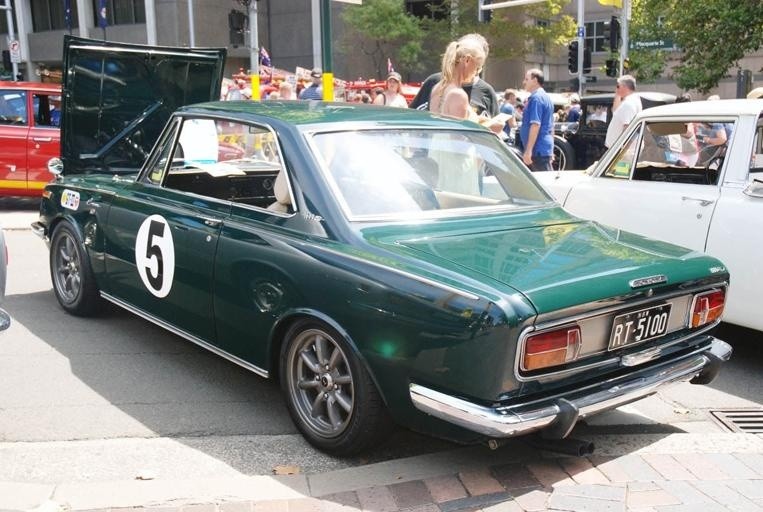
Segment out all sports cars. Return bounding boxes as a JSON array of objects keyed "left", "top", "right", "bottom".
[{"left": 34, "top": 35, "right": 733, "bottom": 456}]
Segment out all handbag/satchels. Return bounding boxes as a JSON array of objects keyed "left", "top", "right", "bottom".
[{"left": 655, "top": 130, "right": 696, "bottom": 152}]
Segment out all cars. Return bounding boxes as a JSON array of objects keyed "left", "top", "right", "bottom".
[
  {"left": 0, "top": 79, "right": 243, "bottom": 199},
  {"left": 510, "top": 92, "right": 677, "bottom": 173}
]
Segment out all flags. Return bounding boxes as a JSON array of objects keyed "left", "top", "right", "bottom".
[
  {"left": 64, "top": 0, "right": 72, "bottom": 30},
  {"left": 259, "top": 46, "right": 271, "bottom": 67},
  {"left": 96, "top": 0, "right": 107, "bottom": 31}
]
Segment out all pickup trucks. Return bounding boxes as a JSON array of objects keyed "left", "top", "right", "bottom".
[{"left": 480, "top": 100, "right": 763, "bottom": 333}]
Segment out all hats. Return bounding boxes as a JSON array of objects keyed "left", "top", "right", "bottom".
[
  {"left": 311, "top": 68, "right": 321, "bottom": 78},
  {"left": 386, "top": 72, "right": 401, "bottom": 81}
]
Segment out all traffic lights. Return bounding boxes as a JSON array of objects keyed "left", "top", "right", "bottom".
[
  {"left": 567, "top": 40, "right": 579, "bottom": 73},
  {"left": 605, "top": 58, "right": 617, "bottom": 78}
]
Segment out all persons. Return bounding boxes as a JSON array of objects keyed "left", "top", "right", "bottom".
[
  {"left": 497, "top": 89, "right": 607, "bottom": 143},
  {"left": 425, "top": 38, "right": 507, "bottom": 197},
  {"left": 604, "top": 74, "right": 642, "bottom": 150},
  {"left": 658, "top": 93, "right": 733, "bottom": 169},
  {"left": 407, "top": 32, "right": 498, "bottom": 197},
  {"left": 520, "top": 68, "right": 555, "bottom": 172},
  {"left": 223, "top": 67, "right": 408, "bottom": 133}
]
